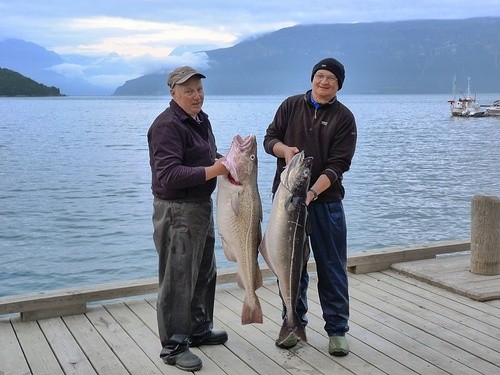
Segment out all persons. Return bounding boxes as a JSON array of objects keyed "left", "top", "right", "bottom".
[
  {"left": 263, "top": 57, "right": 357, "bottom": 355},
  {"left": 147, "top": 66, "right": 229, "bottom": 372}
]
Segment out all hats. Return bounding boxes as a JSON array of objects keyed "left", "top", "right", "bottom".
[
  {"left": 167, "top": 67, "right": 207, "bottom": 88},
  {"left": 311, "top": 58, "right": 345, "bottom": 91}
]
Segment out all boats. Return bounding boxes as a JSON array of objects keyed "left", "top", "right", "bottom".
[{"left": 446, "top": 72, "right": 500, "bottom": 118}]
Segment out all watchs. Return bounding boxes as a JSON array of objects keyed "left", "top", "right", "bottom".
[{"left": 310, "top": 189, "right": 318, "bottom": 200}]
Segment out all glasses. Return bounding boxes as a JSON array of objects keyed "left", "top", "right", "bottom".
[{"left": 314, "top": 73, "right": 338, "bottom": 83}]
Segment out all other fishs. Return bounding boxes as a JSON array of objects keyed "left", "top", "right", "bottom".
[
  {"left": 213, "top": 134, "right": 265, "bottom": 325},
  {"left": 257, "top": 150, "right": 315, "bottom": 345}
]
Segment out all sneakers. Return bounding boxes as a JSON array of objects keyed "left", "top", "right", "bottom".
[
  {"left": 328, "top": 334, "right": 349, "bottom": 355},
  {"left": 175, "top": 354, "right": 202, "bottom": 370},
  {"left": 281, "top": 332, "right": 297, "bottom": 349},
  {"left": 193, "top": 330, "right": 228, "bottom": 347}
]
